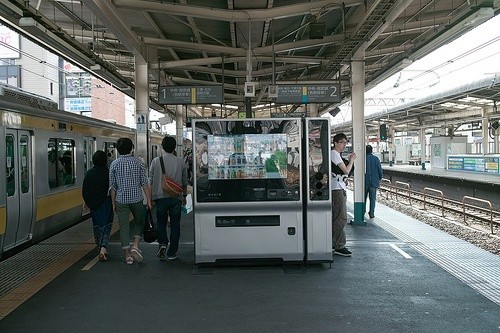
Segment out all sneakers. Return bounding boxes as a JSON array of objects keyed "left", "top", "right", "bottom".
[
  {"left": 332, "top": 246, "right": 335, "bottom": 251},
  {"left": 334, "top": 247, "right": 352, "bottom": 256}
]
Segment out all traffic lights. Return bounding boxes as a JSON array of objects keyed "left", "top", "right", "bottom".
[{"left": 379, "top": 123, "right": 388, "bottom": 141}]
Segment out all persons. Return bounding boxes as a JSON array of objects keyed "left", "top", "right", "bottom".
[
  {"left": 330, "top": 133, "right": 356, "bottom": 256},
  {"left": 365, "top": 145, "right": 382, "bottom": 218},
  {"left": 81, "top": 150, "right": 115, "bottom": 261},
  {"left": 48, "top": 150, "right": 72, "bottom": 187},
  {"left": 149, "top": 136, "right": 187, "bottom": 260},
  {"left": 108, "top": 138, "right": 151, "bottom": 264},
  {"left": 183, "top": 149, "right": 193, "bottom": 186},
  {"left": 255, "top": 152, "right": 262, "bottom": 163}
]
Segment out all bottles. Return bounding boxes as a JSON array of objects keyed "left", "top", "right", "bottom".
[{"left": 211, "top": 140, "right": 266, "bottom": 178}]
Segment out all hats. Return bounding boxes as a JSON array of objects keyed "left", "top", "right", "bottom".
[{"left": 334, "top": 133, "right": 349, "bottom": 142}]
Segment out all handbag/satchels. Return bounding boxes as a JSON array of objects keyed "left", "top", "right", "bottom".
[
  {"left": 144, "top": 207, "right": 157, "bottom": 243},
  {"left": 331, "top": 156, "right": 354, "bottom": 176},
  {"left": 159, "top": 156, "right": 184, "bottom": 196}
]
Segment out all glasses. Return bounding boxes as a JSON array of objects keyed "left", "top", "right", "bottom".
[{"left": 338, "top": 141, "right": 348, "bottom": 144}]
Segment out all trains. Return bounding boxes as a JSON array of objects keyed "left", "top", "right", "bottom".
[{"left": 0, "top": 81, "right": 193, "bottom": 265}]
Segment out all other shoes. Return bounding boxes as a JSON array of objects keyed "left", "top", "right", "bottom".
[
  {"left": 157, "top": 245, "right": 167, "bottom": 258},
  {"left": 370, "top": 215, "right": 375, "bottom": 218},
  {"left": 99, "top": 251, "right": 108, "bottom": 260},
  {"left": 125, "top": 251, "right": 134, "bottom": 264},
  {"left": 131, "top": 245, "right": 144, "bottom": 261},
  {"left": 168, "top": 254, "right": 177, "bottom": 259},
  {"left": 99, "top": 248, "right": 108, "bottom": 253}
]
